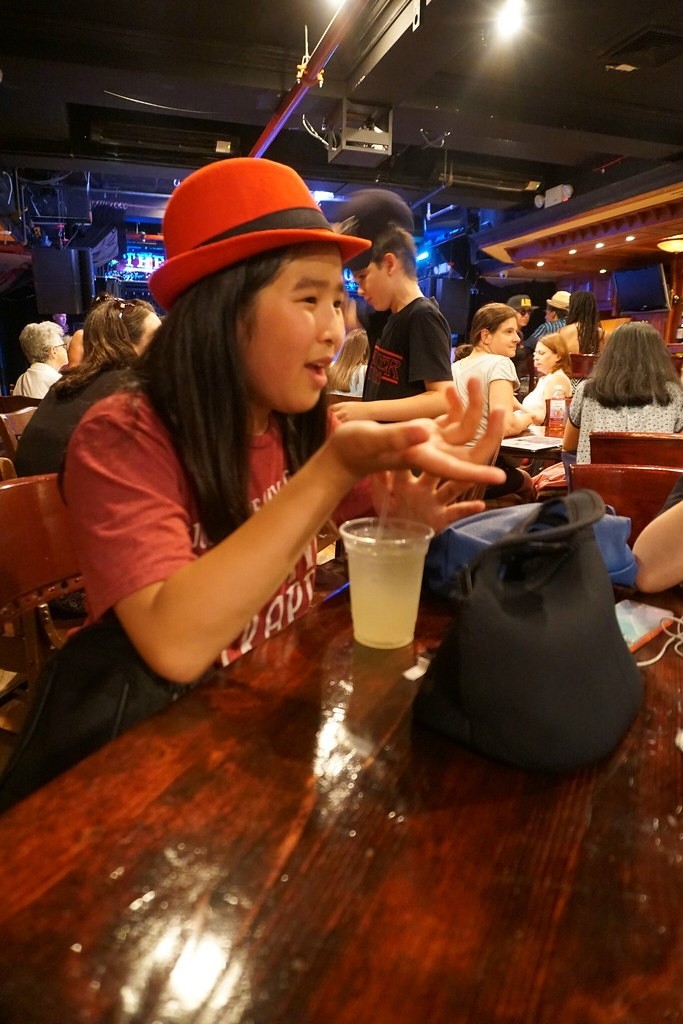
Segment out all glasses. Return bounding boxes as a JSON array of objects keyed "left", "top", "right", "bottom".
[
  {"left": 114, "top": 297, "right": 137, "bottom": 319},
  {"left": 520, "top": 308, "right": 532, "bottom": 316}
]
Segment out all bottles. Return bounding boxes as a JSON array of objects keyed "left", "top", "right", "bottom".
[
  {"left": 549, "top": 385, "right": 566, "bottom": 437},
  {"left": 676, "top": 312, "right": 683, "bottom": 343}
]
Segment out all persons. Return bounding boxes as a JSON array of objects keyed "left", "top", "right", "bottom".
[
  {"left": 632, "top": 472, "right": 683, "bottom": 593},
  {"left": 13, "top": 296, "right": 162, "bottom": 478},
  {"left": 0, "top": 157, "right": 505, "bottom": 815},
  {"left": 330, "top": 189, "right": 458, "bottom": 426},
  {"left": 329, "top": 329, "right": 368, "bottom": 398},
  {"left": 451, "top": 290, "right": 612, "bottom": 504},
  {"left": 563, "top": 321, "right": 683, "bottom": 466},
  {"left": 13, "top": 321, "right": 69, "bottom": 399}
]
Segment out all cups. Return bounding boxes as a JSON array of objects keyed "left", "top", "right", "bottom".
[{"left": 339, "top": 516, "right": 435, "bottom": 649}]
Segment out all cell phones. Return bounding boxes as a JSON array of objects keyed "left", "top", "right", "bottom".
[{"left": 615, "top": 599, "right": 674, "bottom": 653}]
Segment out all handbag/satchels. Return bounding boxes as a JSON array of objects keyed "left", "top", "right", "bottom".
[
  {"left": 408, "top": 488, "right": 645, "bottom": 778},
  {"left": 422, "top": 502, "right": 639, "bottom": 597}
]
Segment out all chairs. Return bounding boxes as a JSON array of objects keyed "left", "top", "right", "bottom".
[
  {"left": 514, "top": 352, "right": 683, "bottom": 553},
  {"left": 0, "top": 395, "right": 85, "bottom": 773}
]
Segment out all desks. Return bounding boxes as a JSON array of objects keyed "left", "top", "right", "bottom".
[
  {"left": 0, "top": 590, "right": 683, "bottom": 1024},
  {"left": 499, "top": 425, "right": 563, "bottom": 476}
]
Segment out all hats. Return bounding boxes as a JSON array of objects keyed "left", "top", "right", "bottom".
[
  {"left": 507, "top": 294, "right": 539, "bottom": 310},
  {"left": 546, "top": 291, "right": 572, "bottom": 309},
  {"left": 146, "top": 157, "right": 373, "bottom": 315}
]
